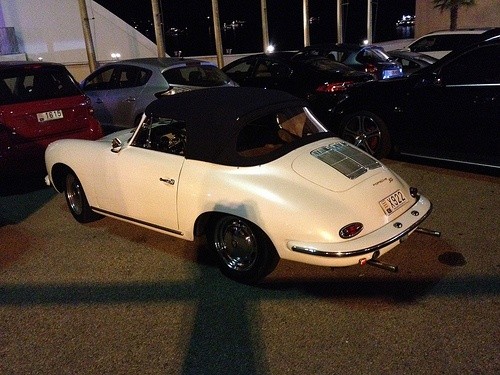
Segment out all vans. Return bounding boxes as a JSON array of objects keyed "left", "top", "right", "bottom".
[{"left": 401, "top": 28, "right": 487, "bottom": 65}]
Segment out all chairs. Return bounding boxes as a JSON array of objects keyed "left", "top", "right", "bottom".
[
  {"left": 32, "top": 73, "right": 61, "bottom": 100},
  {"left": 126, "top": 71, "right": 144, "bottom": 86},
  {"left": 0, "top": 78, "right": 17, "bottom": 102}
]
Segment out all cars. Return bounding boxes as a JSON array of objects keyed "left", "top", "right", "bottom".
[
  {"left": 80, "top": 58, "right": 241, "bottom": 132},
  {"left": 45, "top": 89, "right": 432, "bottom": 283},
  {"left": 220, "top": 53, "right": 377, "bottom": 115},
  {"left": 299, "top": 45, "right": 404, "bottom": 82},
  {"left": 0, "top": 61, "right": 103, "bottom": 190},
  {"left": 333, "top": 27, "right": 500, "bottom": 176},
  {"left": 386, "top": 50, "right": 439, "bottom": 73}
]
